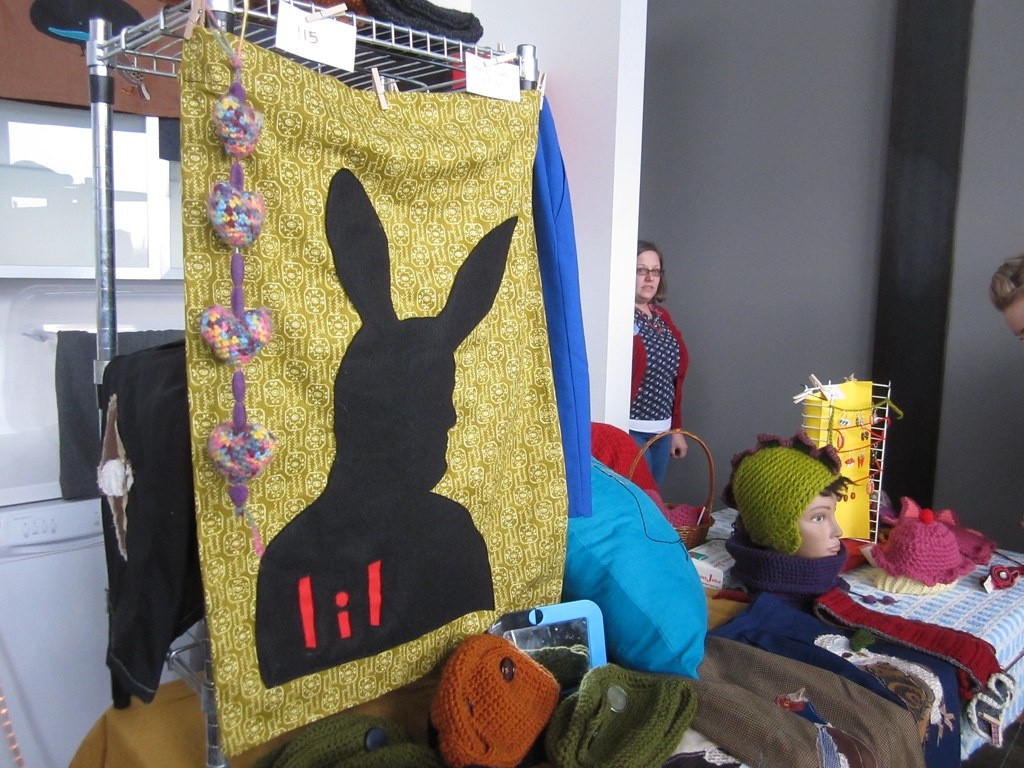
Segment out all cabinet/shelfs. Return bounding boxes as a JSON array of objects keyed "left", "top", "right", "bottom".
[{"left": 87, "top": 0, "right": 539, "bottom": 768}]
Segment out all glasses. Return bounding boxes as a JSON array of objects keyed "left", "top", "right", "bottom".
[{"left": 636, "top": 267, "right": 664, "bottom": 276}]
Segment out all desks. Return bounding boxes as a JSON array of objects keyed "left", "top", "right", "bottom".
[{"left": 67, "top": 506, "right": 1024, "bottom": 768}]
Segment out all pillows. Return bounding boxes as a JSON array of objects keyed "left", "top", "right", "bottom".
[{"left": 565, "top": 457, "right": 706, "bottom": 680}]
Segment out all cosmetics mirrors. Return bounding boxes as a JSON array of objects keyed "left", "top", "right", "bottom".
[{"left": 487, "top": 599, "right": 607, "bottom": 699}]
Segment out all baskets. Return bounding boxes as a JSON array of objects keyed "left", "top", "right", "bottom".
[{"left": 627, "top": 428, "right": 715, "bottom": 549}]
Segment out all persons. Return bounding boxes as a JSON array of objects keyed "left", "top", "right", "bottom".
[
  {"left": 719, "top": 432, "right": 860, "bottom": 595},
  {"left": 629, "top": 239, "right": 690, "bottom": 483},
  {"left": 990, "top": 252, "right": 1024, "bottom": 344}
]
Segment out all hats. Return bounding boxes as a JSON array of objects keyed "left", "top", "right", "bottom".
[
  {"left": 546, "top": 663, "right": 698, "bottom": 768},
  {"left": 252, "top": 707, "right": 438, "bottom": 768},
  {"left": 428, "top": 633, "right": 560, "bottom": 768},
  {"left": 869, "top": 509, "right": 976, "bottom": 587},
  {"left": 722, "top": 428, "right": 843, "bottom": 555}
]
